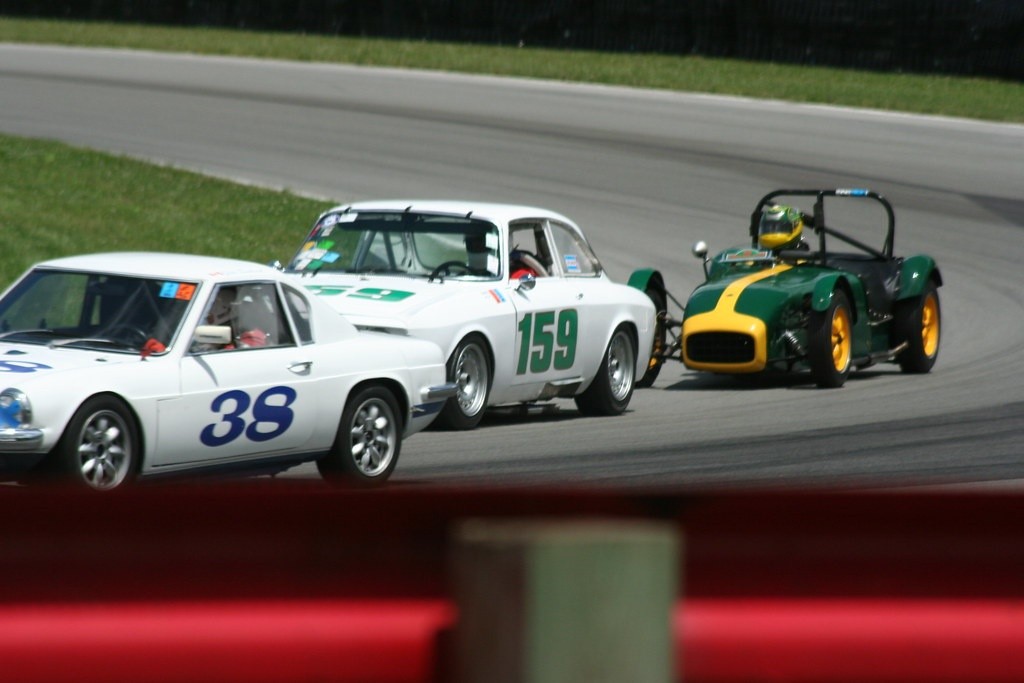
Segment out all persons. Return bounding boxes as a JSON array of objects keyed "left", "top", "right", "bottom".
[
  {"left": 462, "top": 229, "right": 538, "bottom": 278},
  {"left": 140, "top": 285, "right": 264, "bottom": 356},
  {"left": 759, "top": 206, "right": 810, "bottom": 250}
]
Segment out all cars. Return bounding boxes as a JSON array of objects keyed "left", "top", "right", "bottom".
[{"left": 624, "top": 185, "right": 944, "bottom": 390}]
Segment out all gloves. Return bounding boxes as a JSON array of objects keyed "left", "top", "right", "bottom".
[{"left": 142, "top": 338, "right": 165, "bottom": 356}]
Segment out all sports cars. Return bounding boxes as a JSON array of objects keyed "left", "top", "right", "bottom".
[
  {"left": 266, "top": 197, "right": 659, "bottom": 434},
  {"left": 0, "top": 248, "right": 463, "bottom": 506}
]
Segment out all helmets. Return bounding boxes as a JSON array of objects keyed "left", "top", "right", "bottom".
[
  {"left": 463, "top": 231, "right": 513, "bottom": 271},
  {"left": 202, "top": 289, "right": 239, "bottom": 327},
  {"left": 759, "top": 205, "right": 803, "bottom": 251}
]
